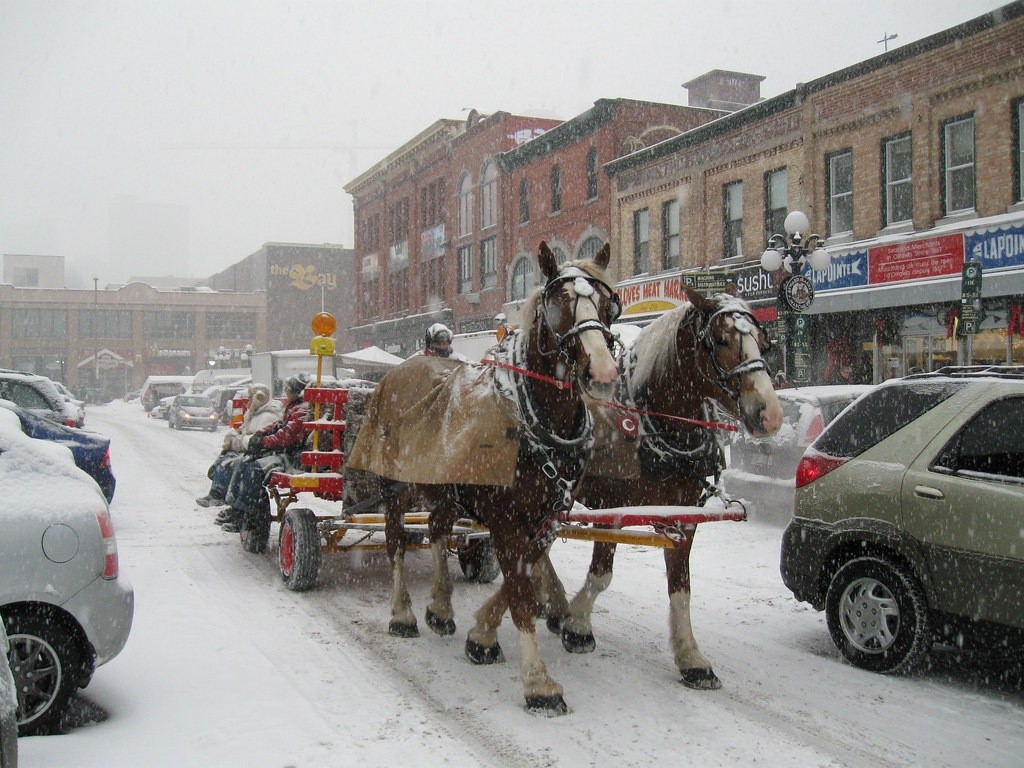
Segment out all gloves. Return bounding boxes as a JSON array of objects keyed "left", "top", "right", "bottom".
[
  {"left": 231, "top": 432, "right": 256, "bottom": 452},
  {"left": 221, "top": 427, "right": 240, "bottom": 451},
  {"left": 243, "top": 433, "right": 266, "bottom": 459}
]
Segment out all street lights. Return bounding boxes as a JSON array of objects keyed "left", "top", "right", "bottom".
[
  {"left": 92, "top": 277, "right": 100, "bottom": 407},
  {"left": 760, "top": 212, "right": 825, "bottom": 388}
]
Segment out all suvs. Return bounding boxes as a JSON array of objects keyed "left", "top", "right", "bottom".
[{"left": 779, "top": 364, "right": 1024, "bottom": 676}]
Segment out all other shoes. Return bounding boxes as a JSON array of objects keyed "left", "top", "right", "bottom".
[
  {"left": 221, "top": 522, "right": 241, "bottom": 533},
  {"left": 196, "top": 489, "right": 226, "bottom": 507},
  {"left": 213, "top": 515, "right": 231, "bottom": 525}
]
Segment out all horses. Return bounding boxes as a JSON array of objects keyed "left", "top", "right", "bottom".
[{"left": 377, "top": 241, "right": 782, "bottom": 719}]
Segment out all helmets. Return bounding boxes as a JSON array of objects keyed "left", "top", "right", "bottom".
[{"left": 425, "top": 322, "right": 455, "bottom": 358}]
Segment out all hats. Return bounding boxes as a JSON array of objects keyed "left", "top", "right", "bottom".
[
  {"left": 283, "top": 372, "right": 311, "bottom": 397},
  {"left": 248, "top": 383, "right": 271, "bottom": 410}
]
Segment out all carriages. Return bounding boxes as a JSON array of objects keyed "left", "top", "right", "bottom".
[{"left": 241, "top": 235, "right": 785, "bottom": 721}]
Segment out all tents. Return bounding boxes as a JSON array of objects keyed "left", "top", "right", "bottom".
[{"left": 337, "top": 344, "right": 406, "bottom": 368}]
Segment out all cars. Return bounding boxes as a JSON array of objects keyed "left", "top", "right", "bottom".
[
  {"left": 167, "top": 394, "right": 219, "bottom": 433},
  {"left": 1, "top": 367, "right": 119, "bottom": 507},
  {"left": 123, "top": 369, "right": 253, "bottom": 425},
  {"left": 722, "top": 385, "right": 877, "bottom": 527},
  {"left": 0, "top": 410, "right": 136, "bottom": 737}
]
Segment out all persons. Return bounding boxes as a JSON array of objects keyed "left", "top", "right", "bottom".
[
  {"left": 406, "top": 322, "right": 470, "bottom": 364},
  {"left": 213, "top": 371, "right": 311, "bottom": 532},
  {"left": 196, "top": 382, "right": 286, "bottom": 508}
]
